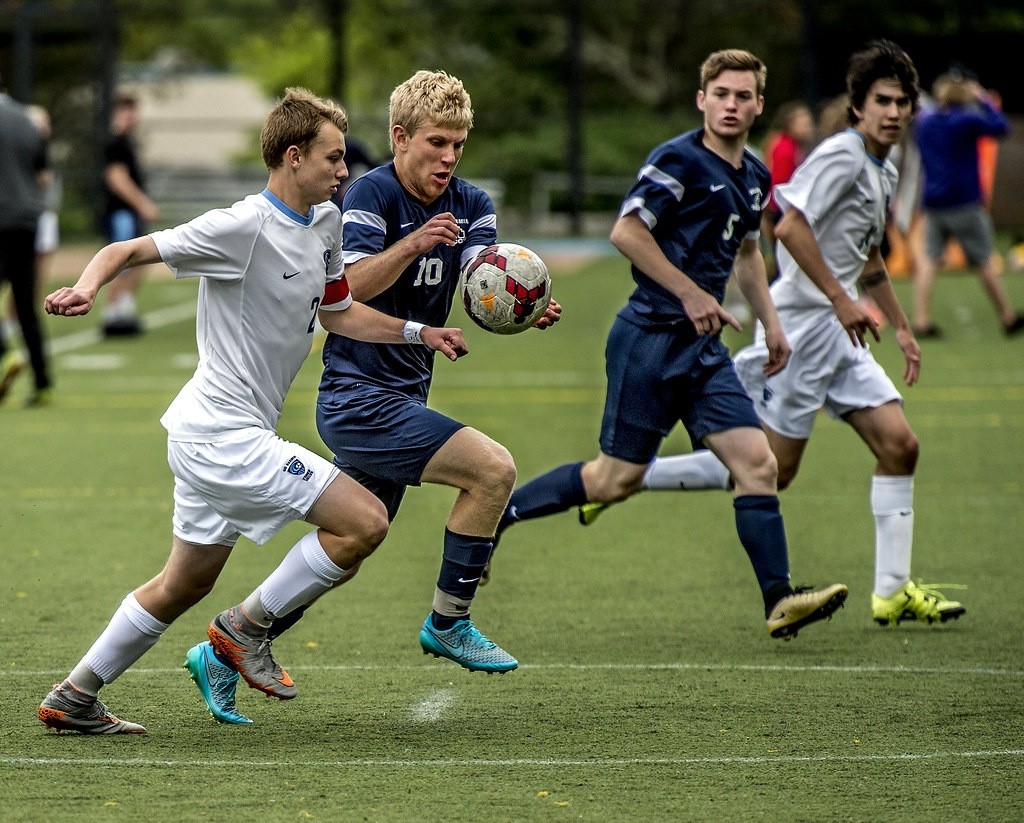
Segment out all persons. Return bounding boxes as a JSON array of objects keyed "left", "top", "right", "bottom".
[
  {"left": 758, "top": 67, "right": 1024, "bottom": 341},
  {"left": 577, "top": 39, "right": 967, "bottom": 629},
  {"left": 182, "top": 67, "right": 563, "bottom": 728},
  {"left": 476, "top": 48, "right": 850, "bottom": 644},
  {"left": 35, "top": 86, "right": 469, "bottom": 736},
  {"left": 102, "top": 98, "right": 159, "bottom": 336},
  {"left": 0, "top": 93, "right": 59, "bottom": 409}
]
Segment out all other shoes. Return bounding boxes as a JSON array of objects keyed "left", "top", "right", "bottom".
[
  {"left": 1005, "top": 316, "right": 1024, "bottom": 337},
  {"left": 916, "top": 325, "right": 942, "bottom": 338},
  {"left": 0, "top": 353, "right": 23, "bottom": 398}
]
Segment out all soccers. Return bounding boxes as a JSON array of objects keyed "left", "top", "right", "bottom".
[{"left": 457, "top": 241, "right": 554, "bottom": 336}]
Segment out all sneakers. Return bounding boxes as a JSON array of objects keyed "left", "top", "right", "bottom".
[
  {"left": 478, "top": 564, "right": 491, "bottom": 586},
  {"left": 869, "top": 578, "right": 969, "bottom": 626},
  {"left": 766, "top": 584, "right": 849, "bottom": 642},
  {"left": 419, "top": 611, "right": 519, "bottom": 676},
  {"left": 578, "top": 503, "right": 601, "bottom": 524},
  {"left": 37, "top": 683, "right": 146, "bottom": 734},
  {"left": 208, "top": 608, "right": 298, "bottom": 700},
  {"left": 183, "top": 640, "right": 253, "bottom": 723}
]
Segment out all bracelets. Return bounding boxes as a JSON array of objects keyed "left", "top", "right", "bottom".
[{"left": 403, "top": 319, "right": 428, "bottom": 344}]
